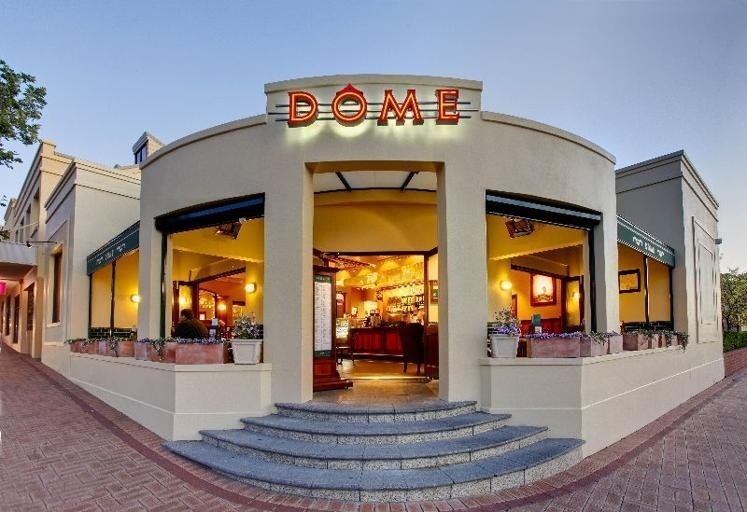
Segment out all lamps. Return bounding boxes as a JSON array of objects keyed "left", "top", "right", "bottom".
[
  {"left": 243, "top": 282, "right": 257, "bottom": 294},
  {"left": 128, "top": 294, "right": 139, "bottom": 303}
]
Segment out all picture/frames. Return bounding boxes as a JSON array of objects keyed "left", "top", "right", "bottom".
[
  {"left": 429, "top": 279, "right": 438, "bottom": 304},
  {"left": 617, "top": 267, "right": 643, "bottom": 294},
  {"left": 529, "top": 274, "right": 558, "bottom": 308},
  {"left": 512, "top": 293, "right": 518, "bottom": 320},
  {"left": 498, "top": 279, "right": 512, "bottom": 292}
]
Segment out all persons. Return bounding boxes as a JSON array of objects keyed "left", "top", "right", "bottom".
[
  {"left": 409, "top": 302, "right": 424, "bottom": 324},
  {"left": 171, "top": 308, "right": 208, "bottom": 338}
]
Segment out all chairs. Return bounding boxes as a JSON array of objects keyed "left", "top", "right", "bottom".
[
  {"left": 398, "top": 320, "right": 427, "bottom": 375},
  {"left": 334, "top": 317, "right": 357, "bottom": 365}
]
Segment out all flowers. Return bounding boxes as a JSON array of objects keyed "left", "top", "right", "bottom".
[
  {"left": 65, "top": 306, "right": 264, "bottom": 368},
  {"left": 488, "top": 305, "right": 690, "bottom": 357}
]
